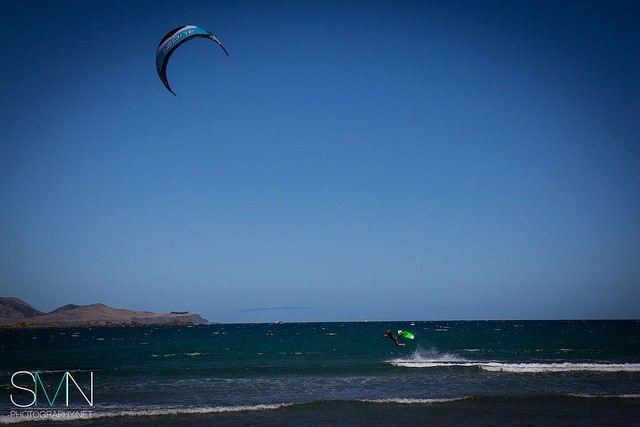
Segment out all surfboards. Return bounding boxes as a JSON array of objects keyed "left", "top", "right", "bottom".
[{"left": 398, "top": 329, "right": 414, "bottom": 339}]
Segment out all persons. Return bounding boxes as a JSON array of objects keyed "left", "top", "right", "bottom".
[{"left": 384, "top": 330, "right": 405, "bottom": 348}]
